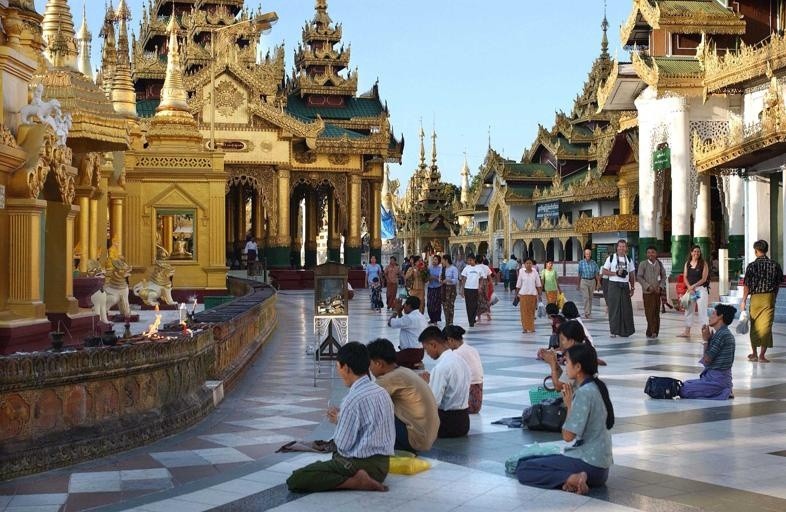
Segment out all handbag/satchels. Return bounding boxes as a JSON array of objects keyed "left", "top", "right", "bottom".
[
  {"left": 644, "top": 375, "right": 684, "bottom": 399},
  {"left": 406, "top": 273, "right": 414, "bottom": 288},
  {"left": 513, "top": 296, "right": 520, "bottom": 306},
  {"left": 529, "top": 387, "right": 561, "bottom": 405},
  {"left": 522, "top": 398, "right": 567, "bottom": 431}
]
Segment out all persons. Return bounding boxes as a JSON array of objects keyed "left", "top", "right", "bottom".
[
  {"left": 677, "top": 300, "right": 736, "bottom": 401},
  {"left": 636, "top": 247, "right": 667, "bottom": 338},
  {"left": 285, "top": 342, "right": 398, "bottom": 496},
  {"left": 326, "top": 337, "right": 442, "bottom": 454},
  {"left": 415, "top": 324, "right": 469, "bottom": 437},
  {"left": 104, "top": 234, "right": 126, "bottom": 271},
  {"left": 739, "top": 237, "right": 785, "bottom": 362},
  {"left": 603, "top": 239, "right": 635, "bottom": 340},
  {"left": 527, "top": 319, "right": 613, "bottom": 429},
  {"left": 677, "top": 245, "right": 710, "bottom": 336},
  {"left": 514, "top": 346, "right": 614, "bottom": 496},
  {"left": 227, "top": 228, "right": 258, "bottom": 271},
  {"left": 171, "top": 232, "right": 193, "bottom": 256},
  {"left": 367, "top": 254, "right": 496, "bottom": 329},
  {"left": 577, "top": 249, "right": 609, "bottom": 317},
  {"left": 500, "top": 254, "right": 561, "bottom": 333},
  {"left": 440, "top": 326, "right": 483, "bottom": 413}
]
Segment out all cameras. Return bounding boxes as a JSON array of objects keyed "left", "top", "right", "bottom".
[{"left": 616, "top": 270, "right": 629, "bottom": 278}]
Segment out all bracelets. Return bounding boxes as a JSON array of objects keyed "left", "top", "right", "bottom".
[{"left": 702, "top": 340, "right": 709, "bottom": 344}]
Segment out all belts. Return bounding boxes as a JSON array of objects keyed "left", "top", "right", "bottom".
[{"left": 582, "top": 277, "right": 596, "bottom": 280}]
